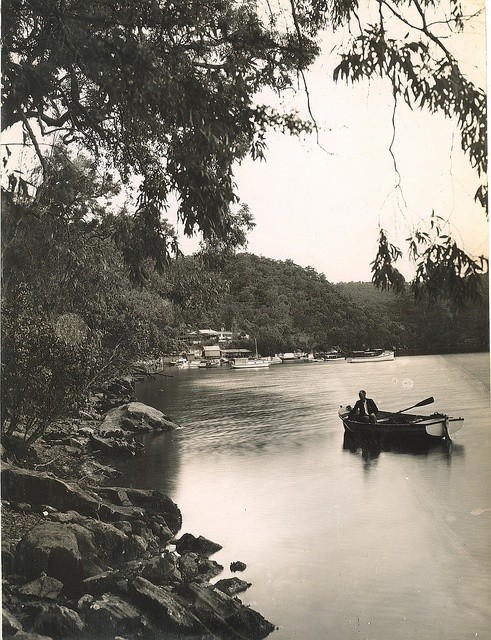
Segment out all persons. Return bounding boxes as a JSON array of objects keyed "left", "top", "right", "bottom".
[{"left": 345, "top": 390, "right": 378, "bottom": 423}]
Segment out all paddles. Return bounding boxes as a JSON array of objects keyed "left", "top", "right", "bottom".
[{"left": 376, "top": 397, "right": 435, "bottom": 420}]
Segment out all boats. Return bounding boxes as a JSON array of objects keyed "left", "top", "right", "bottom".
[
  {"left": 338, "top": 402, "right": 465, "bottom": 437},
  {"left": 348, "top": 350, "right": 395, "bottom": 362},
  {"left": 229, "top": 358, "right": 273, "bottom": 369}
]
